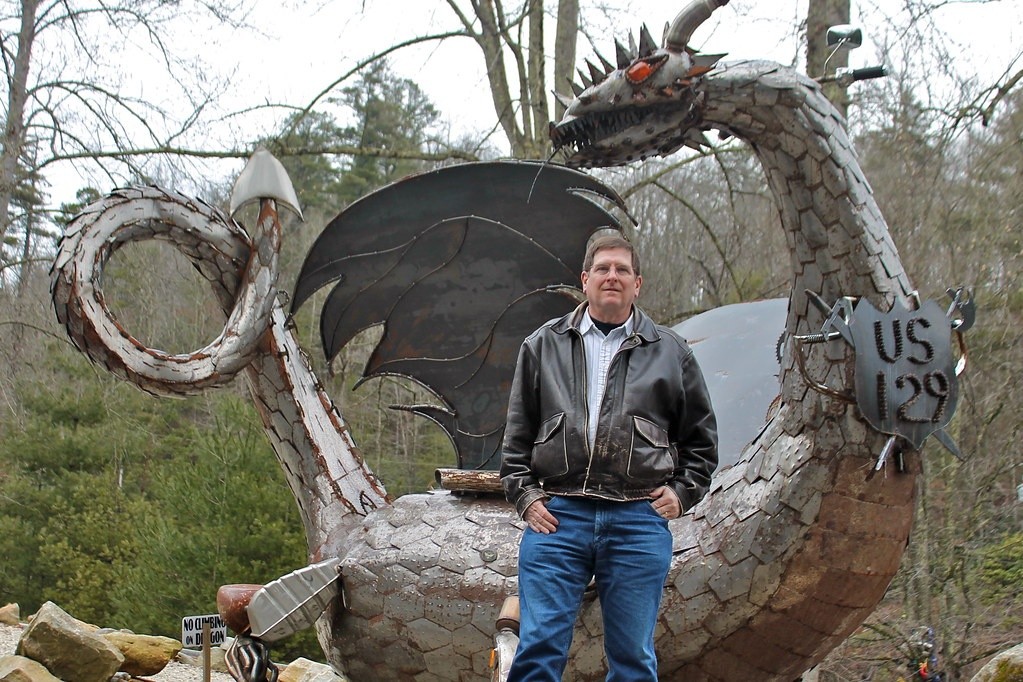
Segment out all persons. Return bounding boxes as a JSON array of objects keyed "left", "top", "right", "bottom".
[{"left": 499, "top": 236, "right": 718, "bottom": 681}]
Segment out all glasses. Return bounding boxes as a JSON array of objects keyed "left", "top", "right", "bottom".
[{"left": 589, "top": 265, "right": 635, "bottom": 278}]
